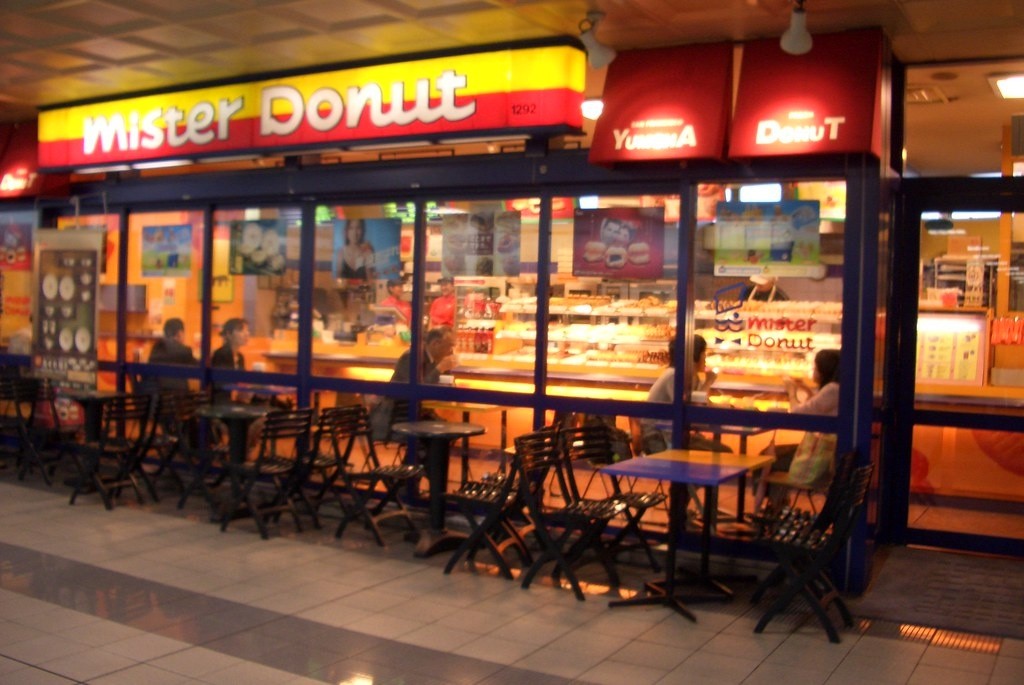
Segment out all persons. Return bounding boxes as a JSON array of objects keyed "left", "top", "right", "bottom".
[
  {"left": 143, "top": 318, "right": 201, "bottom": 439},
  {"left": 427, "top": 276, "right": 456, "bottom": 333},
  {"left": 642, "top": 333, "right": 734, "bottom": 525},
  {"left": 337, "top": 219, "right": 375, "bottom": 279},
  {"left": 754, "top": 348, "right": 843, "bottom": 538},
  {"left": 367, "top": 325, "right": 460, "bottom": 485},
  {"left": 372, "top": 278, "right": 412, "bottom": 329},
  {"left": 212, "top": 317, "right": 295, "bottom": 454},
  {"left": 738, "top": 275, "right": 791, "bottom": 301},
  {"left": 599, "top": 218, "right": 632, "bottom": 249},
  {"left": 7, "top": 312, "right": 38, "bottom": 377}
]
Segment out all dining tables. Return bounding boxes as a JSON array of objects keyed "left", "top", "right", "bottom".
[
  {"left": 655, "top": 419, "right": 773, "bottom": 535},
  {"left": 648, "top": 449, "right": 774, "bottom": 598},
  {"left": 599, "top": 458, "right": 749, "bottom": 623},
  {"left": 222, "top": 382, "right": 323, "bottom": 451},
  {"left": 420, "top": 398, "right": 516, "bottom": 500}
]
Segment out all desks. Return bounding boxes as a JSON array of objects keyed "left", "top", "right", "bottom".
[
  {"left": 60, "top": 389, "right": 129, "bottom": 488},
  {"left": 197, "top": 402, "right": 269, "bottom": 522},
  {"left": 393, "top": 420, "right": 486, "bottom": 556}
]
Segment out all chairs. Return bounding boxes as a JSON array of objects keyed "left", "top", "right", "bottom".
[
  {"left": 219, "top": 408, "right": 313, "bottom": 540},
  {"left": 560, "top": 425, "right": 668, "bottom": 575},
  {"left": 327, "top": 409, "right": 424, "bottom": 548},
  {"left": 273, "top": 403, "right": 362, "bottom": 530},
  {"left": 440, "top": 422, "right": 562, "bottom": 580},
  {"left": 513, "top": 431, "right": 619, "bottom": 600},
  {"left": 751, "top": 463, "right": 876, "bottom": 644},
  {"left": 0, "top": 372, "right": 219, "bottom": 515},
  {"left": 629, "top": 414, "right": 704, "bottom": 521}
]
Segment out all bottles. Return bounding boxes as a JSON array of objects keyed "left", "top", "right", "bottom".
[
  {"left": 454, "top": 326, "right": 494, "bottom": 354},
  {"left": 464, "top": 288, "right": 500, "bottom": 320}
]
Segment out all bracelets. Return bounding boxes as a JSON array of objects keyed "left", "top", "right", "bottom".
[{"left": 788, "top": 394, "right": 797, "bottom": 401}]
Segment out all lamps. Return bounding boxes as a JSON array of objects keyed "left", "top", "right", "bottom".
[
  {"left": 987, "top": 71, "right": 1023, "bottom": 105},
  {"left": 578, "top": 8, "right": 616, "bottom": 70},
  {"left": 780, "top": 0, "right": 812, "bottom": 55}
]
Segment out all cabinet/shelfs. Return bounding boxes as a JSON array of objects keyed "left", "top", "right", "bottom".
[{"left": 495, "top": 292, "right": 844, "bottom": 385}]
